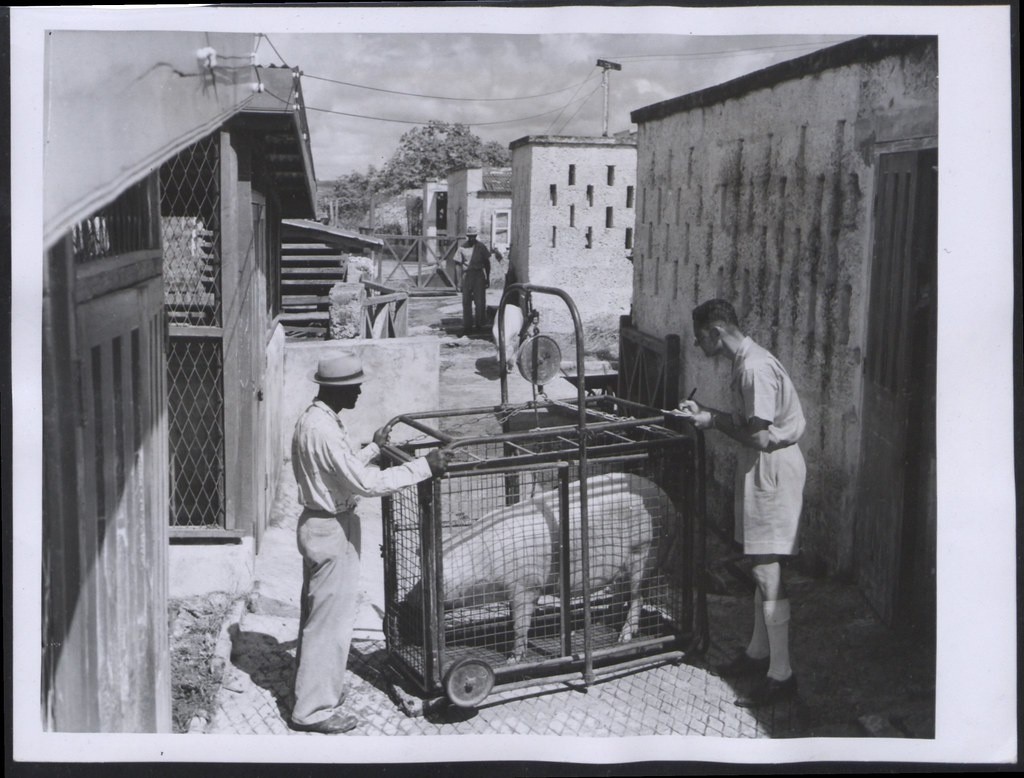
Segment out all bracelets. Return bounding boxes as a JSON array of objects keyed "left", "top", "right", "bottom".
[{"left": 712, "top": 414, "right": 717, "bottom": 428}]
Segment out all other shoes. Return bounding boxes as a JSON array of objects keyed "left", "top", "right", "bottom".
[
  {"left": 288, "top": 713, "right": 358, "bottom": 734},
  {"left": 284, "top": 691, "right": 346, "bottom": 711},
  {"left": 711, "top": 647, "right": 771, "bottom": 678},
  {"left": 734, "top": 673, "right": 798, "bottom": 709}
]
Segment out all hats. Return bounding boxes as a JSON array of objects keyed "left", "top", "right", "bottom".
[
  {"left": 307, "top": 349, "right": 373, "bottom": 385},
  {"left": 466, "top": 227, "right": 478, "bottom": 235}
]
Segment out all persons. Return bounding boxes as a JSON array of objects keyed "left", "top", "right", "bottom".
[
  {"left": 678, "top": 298, "right": 808, "bottom": 709},
  {"left": 452, "top": 226, "right": 491, "bottom": 330},
  {"left": 287, "top": 351, "right": 457, "bottom": 734}
]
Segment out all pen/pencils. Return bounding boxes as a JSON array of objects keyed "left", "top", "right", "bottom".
[{"left": 681, "top": 388, "right": 697, "bottom": 412}]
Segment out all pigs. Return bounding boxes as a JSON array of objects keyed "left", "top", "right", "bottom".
[
  {"left": 406, "top": 470, "right": 681, "bottom": 664},
  {"left": 491, "top": 304, "right": 524, "bottom": 374}
]
